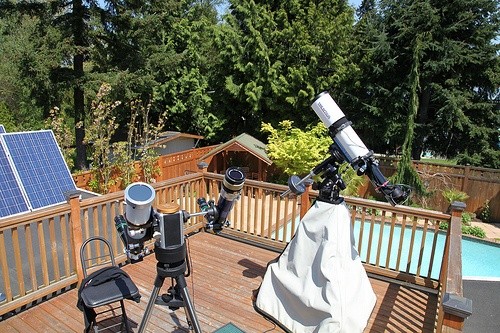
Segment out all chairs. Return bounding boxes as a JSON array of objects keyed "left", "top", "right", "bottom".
[{"left": 79, "top": 235, "right": 141, "bottom": 333}]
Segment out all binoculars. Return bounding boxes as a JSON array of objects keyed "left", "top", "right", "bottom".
[{"left": 115, "top": 165, "right": 246, "bottom": 333}]
